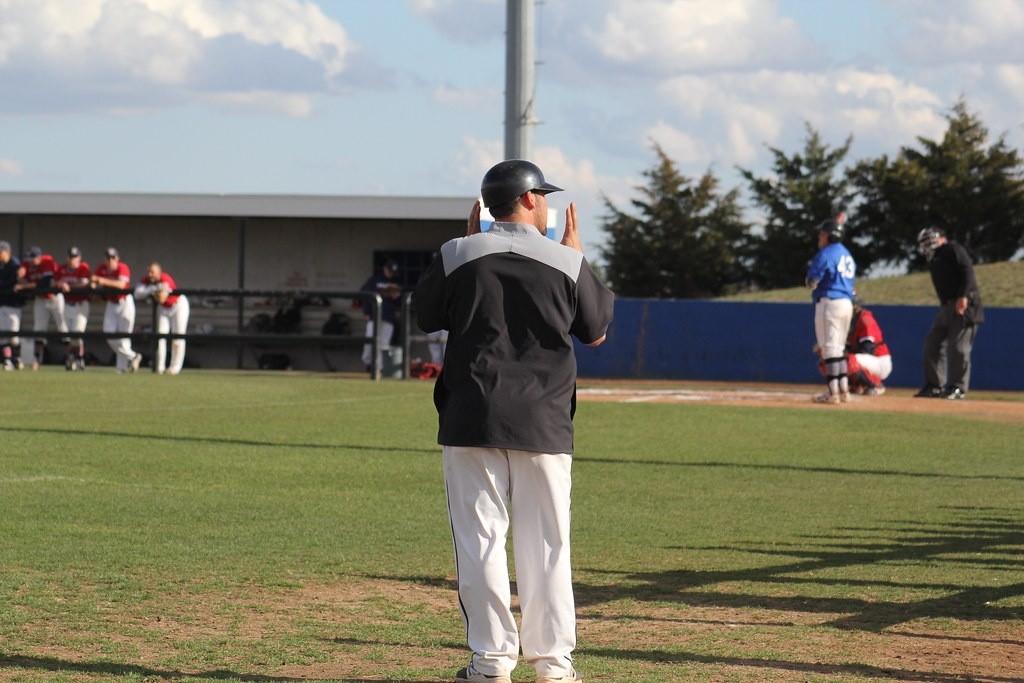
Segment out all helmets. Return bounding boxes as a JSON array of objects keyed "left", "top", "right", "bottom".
[
  {"left": 816, "top": 220, "right": 844, "bottom": 244},
  {"left": 481, "top": 161, "right": 564, "bottom": 207},
  {"left": 917, "top": 225, "right": 944, "bottom": 242},
  {"left": 384, "top": 257, "right": 397, "bottom": 272}
]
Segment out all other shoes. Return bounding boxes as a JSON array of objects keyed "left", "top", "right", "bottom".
[
  {"left": 865, "top": 383, "right": 886, "bottom": 395},
  {"left": 130, "top": 353, "right": 142, "bottom": 372},
  {"left": 811, "top": 389, "right": 851, "bottom": 405},
  {"left": 913, "top": 384, "right": 964, "bottom": 400}
]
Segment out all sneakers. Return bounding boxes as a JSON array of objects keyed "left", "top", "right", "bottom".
[
  {"left": 535, "top": 669, "right": 582, "bottom": 683},
  {"left": 455, "top": 661, "right": 511, "bottom": 683}
]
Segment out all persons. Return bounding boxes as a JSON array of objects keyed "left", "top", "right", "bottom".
[
  {"left": 358, "top": 258, "right": 404, "bottom": 380},
  {"left": 0, "top": 240, "right": 190, "bottom": 376},
  {"left": 412, "top": 160, "right": 614, "bottom": 683},
  {"left": 913, "top": 227, "right": 983, "bottom": 399},
  {"left": 804, "top": 217, "right": 893, "bottom": 404}
]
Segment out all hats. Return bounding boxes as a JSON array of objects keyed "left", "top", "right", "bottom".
[
  {"left": 0, "top": 241, "right": 10, "bottom": 251},
  {"left": 26, "top": 245, "right": 41, "bottom": 261},
  {"left": 104, "top": 246, "right": 117, "bottom": 256},
  {"left": 66, "top": 246, "right": 80, "bottom": 256}
]
